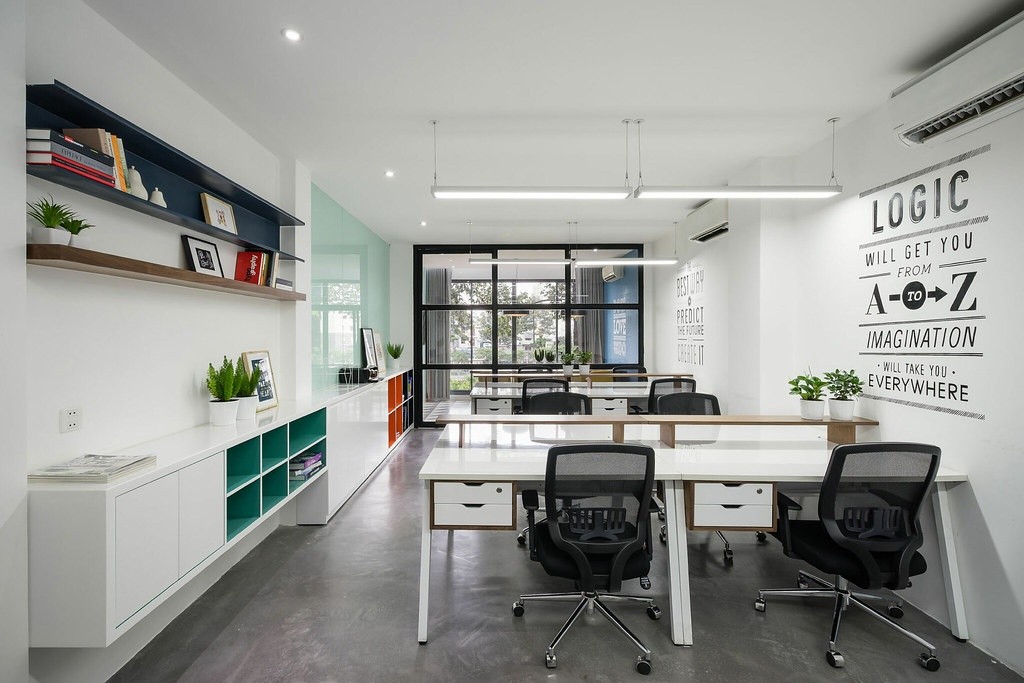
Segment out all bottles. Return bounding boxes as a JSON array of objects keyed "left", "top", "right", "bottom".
[
  {"left": 128, "top": 165, "right": 148, "bottom": 201},
  {"left": 149, "top": 187, "right": 167, "bottom": 209}
]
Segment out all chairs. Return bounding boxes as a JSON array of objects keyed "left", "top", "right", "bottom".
[{"left": 519, "top": 365, "right": 949, "bottom": 675}]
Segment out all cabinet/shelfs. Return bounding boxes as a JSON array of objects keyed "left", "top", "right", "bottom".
[
  {"left": 29, "top": 370, "right": 418, "bottom": 649},
  {"left": 25, "top": 80, "right": 306, "bottom": 301}
]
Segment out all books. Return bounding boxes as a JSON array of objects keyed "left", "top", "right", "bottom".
[
  {"left": 26, "top": 129, "right": 115, "bottom": 187},
  {"left": 62, "top": 129, "right": 132, "bottom": 195},
  {"left": 290, "top": 451, "right": 322, "bottom": 481},
  {"left": 408, "top": 378, "right": 412, "bottom": 397},
  {"left": 26, "top": 454, "right": 158, "bottom": 484},
  {"left": 275, "top": 278, "right": 294, "bottom": 291},
  {"left": 235, "top": 248, "right": 280, "bottom": 288},
  {"left": 404, "top": 407, "right": 406, "bottom": 430}
]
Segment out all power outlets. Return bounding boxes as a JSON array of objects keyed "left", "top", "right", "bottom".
[{"left": 59, "top": 407, "right": 84, "bottom": 431}]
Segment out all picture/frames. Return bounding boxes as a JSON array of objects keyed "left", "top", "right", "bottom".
[
  {"left": 181, "top": 236, "right": 225, "bottom": 277},
  {"left": 241, "top": 351, "right": 279, "bottom": 413}
]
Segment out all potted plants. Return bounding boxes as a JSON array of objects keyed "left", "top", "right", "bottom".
[
  {"left": 534, "top": 349, "right": 592, "bottom": 374},
  {"left": 790, "top": 374, "right": 826, "bottom": 420},
  {"left": 829, "top": 372, "right": 859, "bottom": 420},
  {"left": 207, "top": 357, "right": 259, "bottom": 425},
  {"left": 29, "top": 196, "right": 89, "bottom": 247},
  {"left": 388, "top": 343, "right": 404, "bottom": 368}
]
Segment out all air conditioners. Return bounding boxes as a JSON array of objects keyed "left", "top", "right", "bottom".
[
  {"left": 686, "top": 199, "right": 728, "bottom": 243},
  {"left": 602, "top": 266, "right": 623, "bottom": 282},
  {"left": 887, "top": 11, "right": 1024, "bottom": 147}
]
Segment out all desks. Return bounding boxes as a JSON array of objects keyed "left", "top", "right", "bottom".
[
  {"left": 419, "top": 414, "right": 970, "bottom": 643},
  {"left": 470, "top": 381, "right": 691, "bottom": 415}
]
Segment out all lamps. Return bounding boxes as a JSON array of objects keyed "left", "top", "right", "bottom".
[
  {"left": 430, "top": 117, "right": 842, "bottom": 198},
  {"left": 504, "top": 310, "right": 586, "bottom": 317},
  {"left": 467, "top": 222, "right": 678, "bottom": 265}
]
